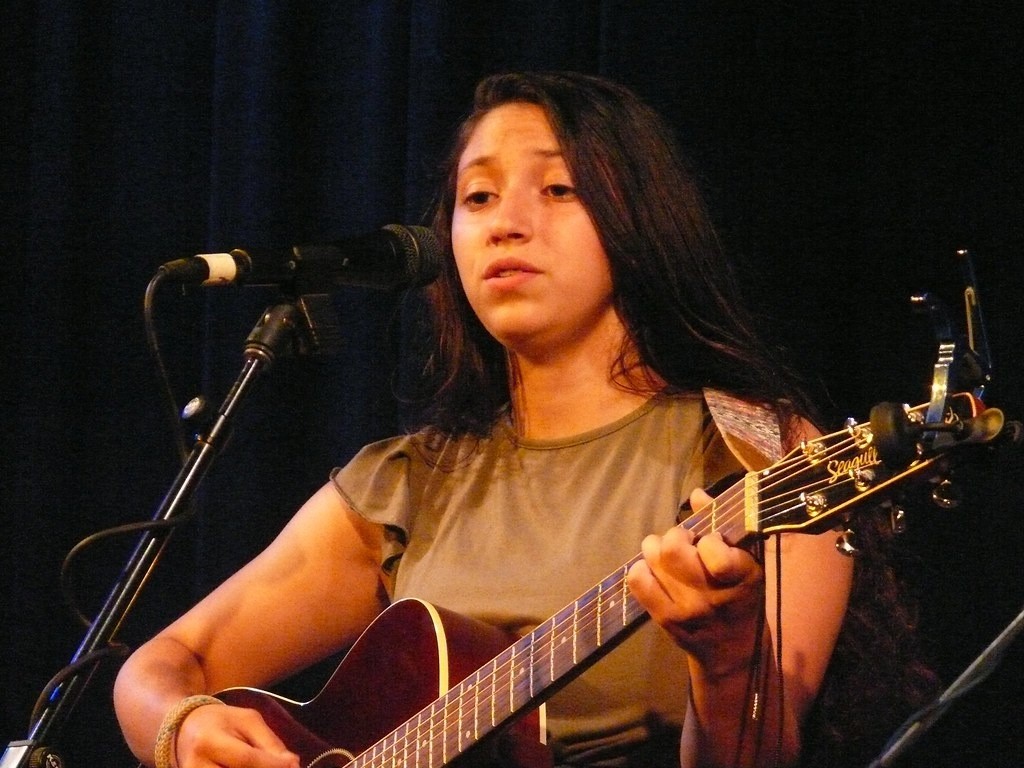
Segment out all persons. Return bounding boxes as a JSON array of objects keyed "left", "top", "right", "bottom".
[{"left": 114, "top": 75, "right": 853, "bottom": 768}]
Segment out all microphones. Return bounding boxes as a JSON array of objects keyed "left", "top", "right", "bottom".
[{"left": 160, "top": 224, "right": 444, "bottom": 295}]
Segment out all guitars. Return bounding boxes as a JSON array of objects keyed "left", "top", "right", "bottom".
[{"left": 137, "top": 246, "right": 1023, "bottom": 768}]
